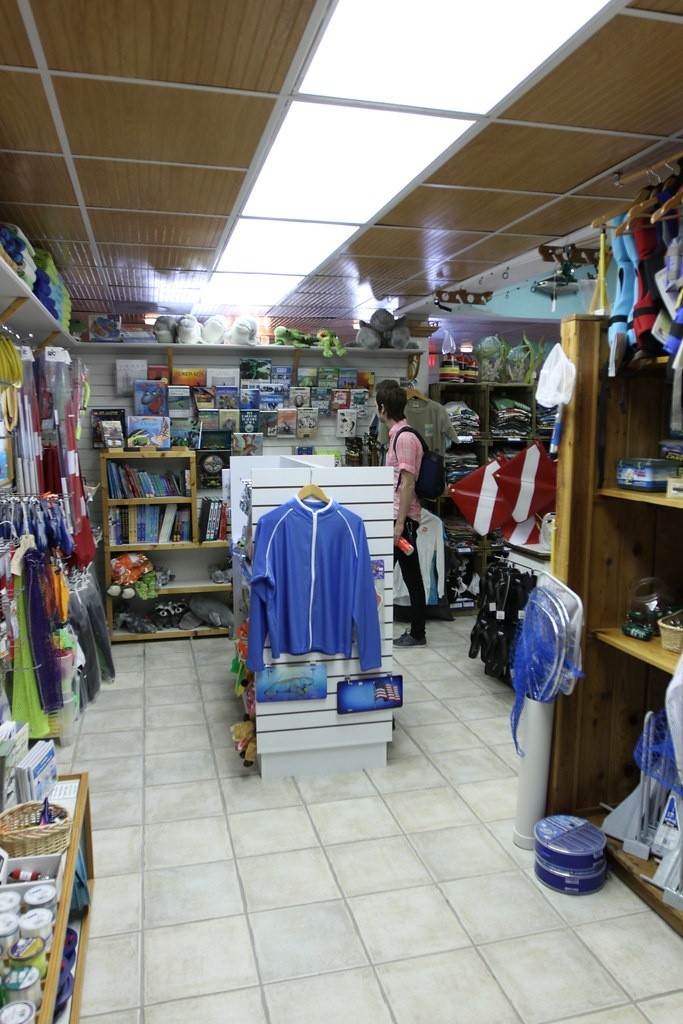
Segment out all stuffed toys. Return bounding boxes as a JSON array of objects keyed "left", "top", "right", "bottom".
[
  {"left": 274, "top": 326, "right": 347, "bottom": 358},
  {"left": 110, "top": 596, "right": 234, "bottom": 639},
  {"left": 153, "top": 314, "right": 260, "bottom": 346},
  {"left": 231, "top": 722, "right": 255, "bottom": 767},
  {"left": 107, "top": 553, "right": 170, "bottom": 600},
  {"left": 209, "top": 565, "right": 233, "bottom": 583},
  {"left": 346, "top": 309, "right": 419, "bottom": 350}
]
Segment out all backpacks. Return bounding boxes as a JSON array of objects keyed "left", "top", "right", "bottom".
[{"left": 393, "top": 426, "right": 446, "bottom": 500}]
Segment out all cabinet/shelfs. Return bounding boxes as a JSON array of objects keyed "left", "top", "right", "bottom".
[
  {"left": 430, "top": 382, "right": 549, "bottom": 617},
  {"left": 570, "top": 317, "right": 683, "bottom": 938},
  {"left": 100, "top": 450, "right": 233, "bottom": 643},
  {"left": 0, "top": 772, "right": 95, "bottom": 1024}
]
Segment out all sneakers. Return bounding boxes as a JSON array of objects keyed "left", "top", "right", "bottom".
[{"left": 392, "top": 628, "right": 428, "bottom": 648}]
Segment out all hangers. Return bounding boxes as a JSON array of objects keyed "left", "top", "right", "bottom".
[
  {"left": 627, "top": 157, "right": 677, "bottom": 220},
  {"left": 406, "top": 380, "right": 428, "bottom": 402},
  {"left": 650, "top": 190, "right": 683, "bottom": 224},
  {"left": 297, "top": 465, "right": 330, "bottom": 503},
  {"left": 614, "top": 161, "right": 661, "bottom": 237},
  {"left": 590, "top": 164, "right": 656, "bottom": 229}
]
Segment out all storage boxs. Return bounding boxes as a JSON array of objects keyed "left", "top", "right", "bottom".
[
  {"left": 122, "top": 332, "right": 156, "bottom": 343},
  {"left": 88, "top": 315, "right": 122, "bottom": 342},
  {"left": 0, "top": 853, "right": 65, "bottom": 905}
]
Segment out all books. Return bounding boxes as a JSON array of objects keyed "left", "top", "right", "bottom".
[
  {"left": 0, "top": 720, "right": 57, "bottom": 811},
  {"left": 91, "top": 358, "right": 375, "bottom": 456},
  {"left": 107, "top": 460, "right": 191, "bottom": 499},
  {"left": 108, "top": 504, "right": 191, "bottom": 546},
  {"left": 198, "top": 496, "right": 231, "bottom": 544}
]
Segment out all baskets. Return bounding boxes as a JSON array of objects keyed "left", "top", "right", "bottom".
[{"left": 0, "top": 801, "right": 73, "bottom": 858}]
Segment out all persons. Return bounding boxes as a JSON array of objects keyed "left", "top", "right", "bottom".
[
  {"left": 376, "top": 380, "right": 427, "bottom": 648},
  {"left": 299, "top": 418, "right": 306, "bottom": 429},
  {"left": 309, "top": 419, "right": 315, "bottom": 428},
  {"left": 295, "top": 395, "right": 304, "bottom": 408}
]
